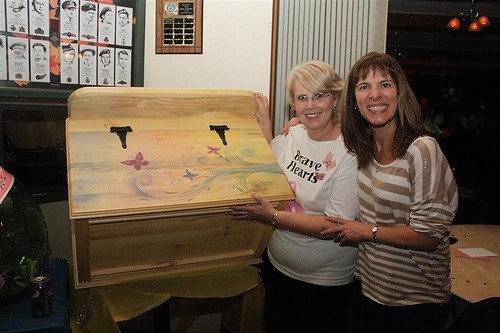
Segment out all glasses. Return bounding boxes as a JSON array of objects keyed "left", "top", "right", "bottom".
[{"left": 293, "top": 92, "right": 331, "bottom": 104}]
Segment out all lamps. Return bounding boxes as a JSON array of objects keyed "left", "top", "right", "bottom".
[
  {"left": 445, "top": 0, "right": 491, "bottom": 32},
  {"left": 387, "top": 31, "right": 404, "bottom": 58}
]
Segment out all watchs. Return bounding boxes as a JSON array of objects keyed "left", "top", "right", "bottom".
[
  {"left": 371, "top": 224, "right": 381, "bottom": 244},
  {"left": 272, "top": 210, "right": 278, "bottom": 226}
]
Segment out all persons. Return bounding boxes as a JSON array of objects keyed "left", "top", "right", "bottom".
[
  {"left": 225, "top": 60, "right": 359, "bottom": 333},
  {"left": 279, "top": 53, "right": 458, "bottom": 333}
]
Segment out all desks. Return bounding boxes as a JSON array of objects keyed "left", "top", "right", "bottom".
[
  {"left": 120, "top": 265, "right": 264, "bottom": 333},
  {"left": 447, "top": 224, "right": 500, "bottom": 333},
  {"left": 0, "top": 259, "right": 71, "bottom": 333}
]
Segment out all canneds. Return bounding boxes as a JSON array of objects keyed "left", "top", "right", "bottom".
[{"left": 31, "top": 277, "right": 52, "bottom": 318}]
